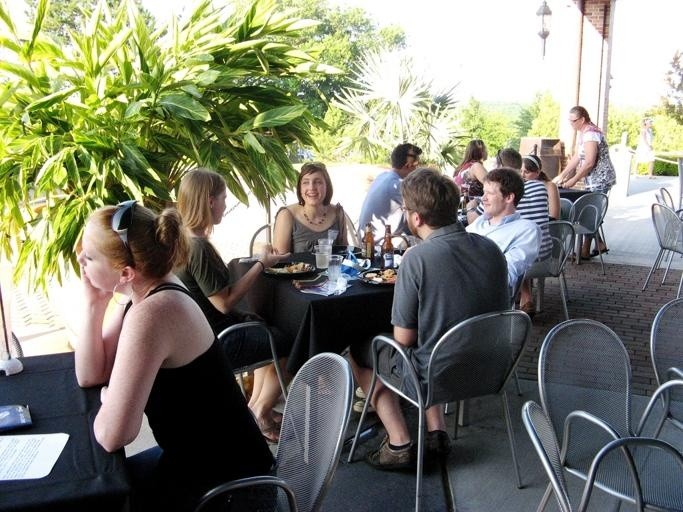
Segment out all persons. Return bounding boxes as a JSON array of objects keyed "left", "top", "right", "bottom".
[
  {"left": 272, "top": 161, "right": 348, "bottom": 396},
  {"left": 358, "top": 142, "right": 421, "bottom": 235},
  {"left": 169, "top": 168, "right": 283, "bottom": 444},
  {"left": 72, "top": 200, "right": 278, "bottom": 511},
  {"left": 551, "top": 106, "right": 616, "bottom": 261},
  {"left": 451, "top": 138, "right": 561, "bottom": 316},
  {"left": 343, "top": 167, "right": 506, "bottom": 471}
]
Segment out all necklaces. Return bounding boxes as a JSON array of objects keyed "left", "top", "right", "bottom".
[{"left": 302, "top": 205, "right": 326, "bottom": 226}]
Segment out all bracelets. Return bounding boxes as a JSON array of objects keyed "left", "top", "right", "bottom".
[{"left": 256, "top": 261, "right": 264, "bottom": 272}]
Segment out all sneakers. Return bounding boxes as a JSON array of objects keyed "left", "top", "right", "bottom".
[
  {"left": 354, "top": 398, "right": 377, "bottom": 414},
  {"left": 356, "top": 387, "right": 367, "bottom": 400},
  {"left": 516, "top": 300, "right": 536, "bottom": 320},
  {"left": 364, "top": 433, "right": 416, "bottom": 471},
  {"left": 423, "top": 429, "right": 453, "bottom": 464}
]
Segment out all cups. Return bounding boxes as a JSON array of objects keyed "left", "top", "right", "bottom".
[{"left": 313, "top": 237, "right": 344, "bottom": 283}]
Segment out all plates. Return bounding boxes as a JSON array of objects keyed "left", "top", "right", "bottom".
[
  {"left": 359, "top": 269, "right": 396, "bottom": 288},
  {"left": 310, "top": 244, "right": 362, "bottom": 257},
  {"left": 263, "top": 261, "right": 315, "bottom": 278}
]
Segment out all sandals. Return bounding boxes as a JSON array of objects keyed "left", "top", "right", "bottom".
[
  {"left": 270, "top": 409, "right": 284, "bottom": 424},
  {"left": 257, "top": 422, "right": 281, "bottom": 443}
]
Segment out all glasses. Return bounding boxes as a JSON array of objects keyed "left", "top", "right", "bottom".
[
  {"left": 569, "top": 118, "right": 581, "bottom": 123},
  {"left": 301, "top": 161, "right": 326, "bottom": 171},
  {"left": 110, "top": 199, "right": 138, "bottom": 270}
]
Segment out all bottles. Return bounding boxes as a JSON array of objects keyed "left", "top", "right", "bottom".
[
  {"left": 380, "top": 225, "right": 394, "bottom": 271},
  {"left": 461, "top": 189, "right": 470, "bottom": 216},
  {"left": 361, "top": 223, "right": 374, "bottom": 263}
]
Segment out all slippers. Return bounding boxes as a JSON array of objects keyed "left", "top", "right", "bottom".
[
  {"left": 588, "top": 246, "right": 609, "bottom": 258},
  {"left": 567, "top": 253, "right": 591, "bottom": 262}
]
